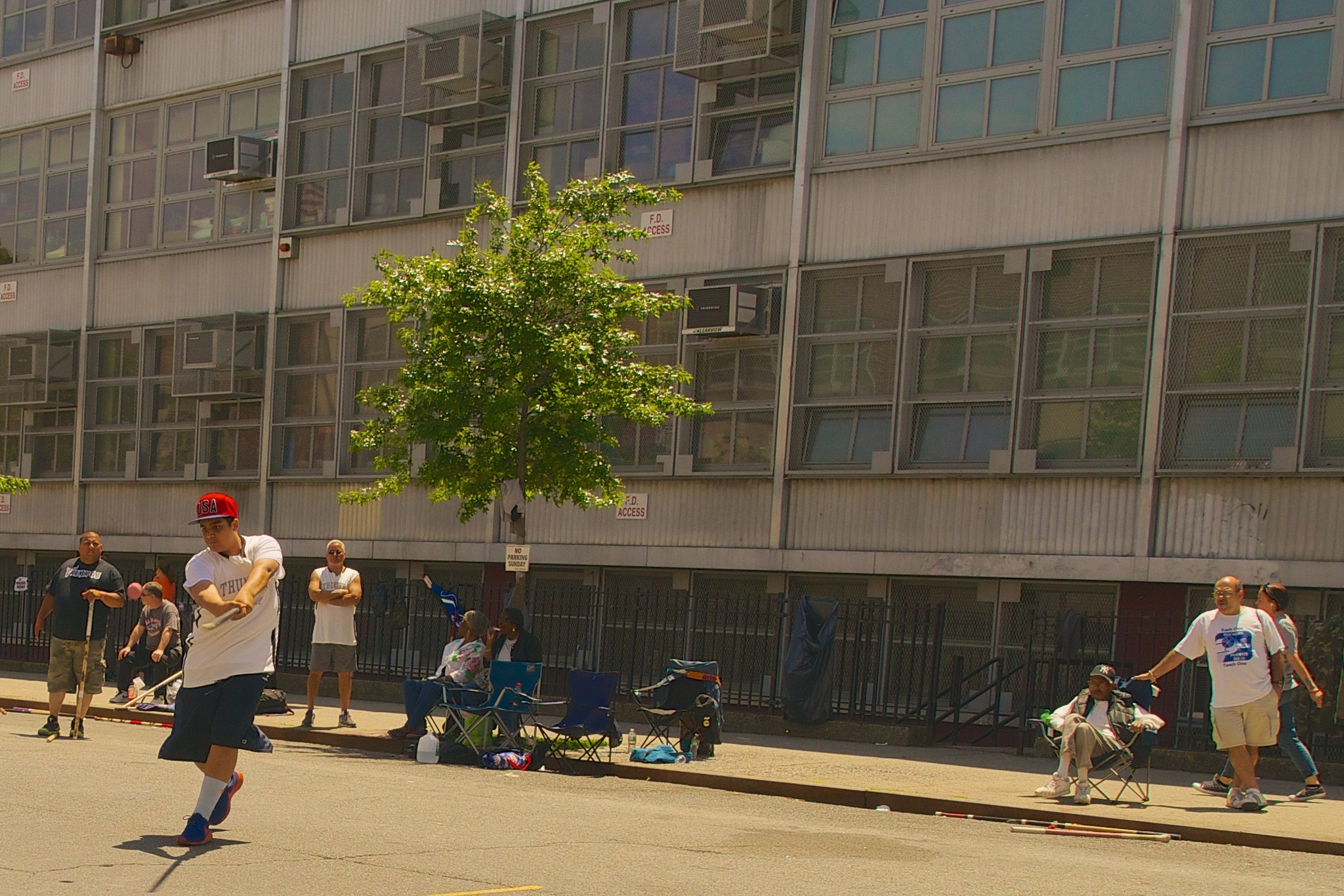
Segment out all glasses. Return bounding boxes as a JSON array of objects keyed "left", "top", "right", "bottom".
[
  {"left": 1212, "top": 591, "right": 1235, "bottom": 598},
  {"left": 328, "top": 551, "right": 343, "bottom": 555},
  {"left": 142, "top": 592, "right": 151, "bottom": 597},
  {"left": 1087, "top": 680, "right": 1106, "bottom": 688},
  {"left": 1265, "top": 582, "right": 1270, "bottom": 595}
]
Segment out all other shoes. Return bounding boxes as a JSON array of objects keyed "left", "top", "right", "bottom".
[
  {"left": 388, "top": 726, "right": 406, "bottom": 739},
  {"left": 405, "top": 727, "right": 427, "bottom": 738}
]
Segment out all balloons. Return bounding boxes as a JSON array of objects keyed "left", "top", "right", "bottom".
[{"left": 126, "top": 582, "right": 142, "bottom": 599}]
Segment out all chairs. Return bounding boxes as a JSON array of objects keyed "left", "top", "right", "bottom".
[
  {"left": 622, "top": 659, "right": 719, "bottom": 756},
  {"left": 1027, "top": 674, "right": 1158, "bottom": 805},
  {"left": 521, "top": 669, "right": 620, "bottom": 776},
  {"left": 426, "top": 661, "right": 491, "bottom": 737},
  {"left": 438, "top": 660, "right": 543, "bottom": 768}
]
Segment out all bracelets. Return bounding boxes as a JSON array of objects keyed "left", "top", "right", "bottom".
[
  {"left": 1148, "top": 670, "right": 1156, "bottom": 681},
  {"left": 1307, "top": 688, "right": 1322, "bottom": 694}
]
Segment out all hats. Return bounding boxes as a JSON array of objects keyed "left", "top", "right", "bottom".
[
  {"left": 1090, "top": 664, "right": 1116, "bottom": 684},
  {"left": 188, "top": 494, "right": 238, "bottom": 525}
]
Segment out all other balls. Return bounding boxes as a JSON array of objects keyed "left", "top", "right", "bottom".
[{"left": 127, "top": 582, "right": 143, "bottom": 599}]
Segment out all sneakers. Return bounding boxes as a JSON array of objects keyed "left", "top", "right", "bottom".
[
  {"left": 1240, "top": 791, "right": 1267, "bottom": 811},
  {"left": 176, "top": 812, "right": 212, "bottom": 845},
  {"left": 1225, "top": 789, "right": 1243, "bottom": 808},
  {"left": 70, "top": 718, "right": 84, "bottom": 737},
  {"left": 1192, "top": 774, "right": 1227, "bottom": 796},
  {"left": 1288, "top": 786, "right": 1325, "bottom": 801},
  {"left": 38, "top": 717, "right": 61, "bottom": 735},
  {"left": 338, "top": 712, "right": 356, "bottom": 728},
  {"left": 302, "top": 712, "right": 315, "bottom": 726},
  {"left": 1035, "top": 772, "right": 1070, "bottom": 797},
  {"left": 209, "top": 771, "right": 243, "bottom": 826},
  {"left": 110, "top": 690, "right": 129, "bottom": 703},
  {"left": 1073, "top": 782, "right": 1091, "bottom": 804},
  {"left": 151, "top": 695, "right": 165, "bottom": 704}
]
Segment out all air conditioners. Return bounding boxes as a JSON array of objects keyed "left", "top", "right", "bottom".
[
  {"left": 183, "top": 328, "right": 254, "bottom": 371},
  {"left": 698, "top": 0, "right": 789, "bottom": 43},
  {"left": 204, "top": 135, "right": 270, "bottom": 182},
  {"left": 420, "top": 35, "right": 504, "bottom": 93},
  {"left": 682, "top": 284, "right": 769, "bottom": 338},
  {"left": 7, "top": 344, "right": 73, "bottom": 382}
]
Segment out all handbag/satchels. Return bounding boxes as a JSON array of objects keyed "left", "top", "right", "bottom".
[
  {"left": 483, "top": 751, "right": 532, "bottom": 770},
  {"left": 254, "top": 688, "right": 294, "bottom": 716},
  {"left": 630, "top": 743, "right": 691, "bottom": 763}
]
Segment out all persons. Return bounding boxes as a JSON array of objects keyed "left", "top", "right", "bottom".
[
  {"left": 34, "top": 531, "right": 126, "bottom": 737},
  {"left": 106, "top": 582, "right": 182, "bottom": 706},
  {"left": 1192, "top": 581, "right": 1327, "bottom": 804},
  {"left": 1035, "top": 663, "right": 1166, "bottom": 804},
  {"left": 1133, "top": 576, "right": 1287, "bottom": 811},
  {"left": 156, "top": 493, "right": 286, "bottom": 846},
  {"left": 443, "top": 607, "right": 543, "bottom": 734},
  {"left": 387, "top": 610, "right": 491, "bottom": 740},
  {"left": 153, "top": 559, "right": 176, "bottom": 603},
  {"left": 301, "top": 539, "right": 363, "bottom": 726}
]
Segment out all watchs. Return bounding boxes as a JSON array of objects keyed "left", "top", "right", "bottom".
[{"left": 1271, "top": 680, "right": 1283, "bottom": 686}]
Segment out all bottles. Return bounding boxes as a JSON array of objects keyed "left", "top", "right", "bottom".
[
  {"left": 689, "top": 734, "right": 700, "bottom": 759},
  {"left": 1040, "top": 709, "right": 1050, "bottom": 725},
  {"left": 675, "top": 752, "right": 694, "bottom": 765},
  {"left": 514, "top": 683, "right": 521, "bottom": 693},
  {"left": 416, "top": 732, "right": 439, "bottom": 763},
  {"left": 128, "top": 682, "right": 137, "bottom": 707},
  {"left": 628, "top": 729, "right": 636, "bottom": 754}
]
